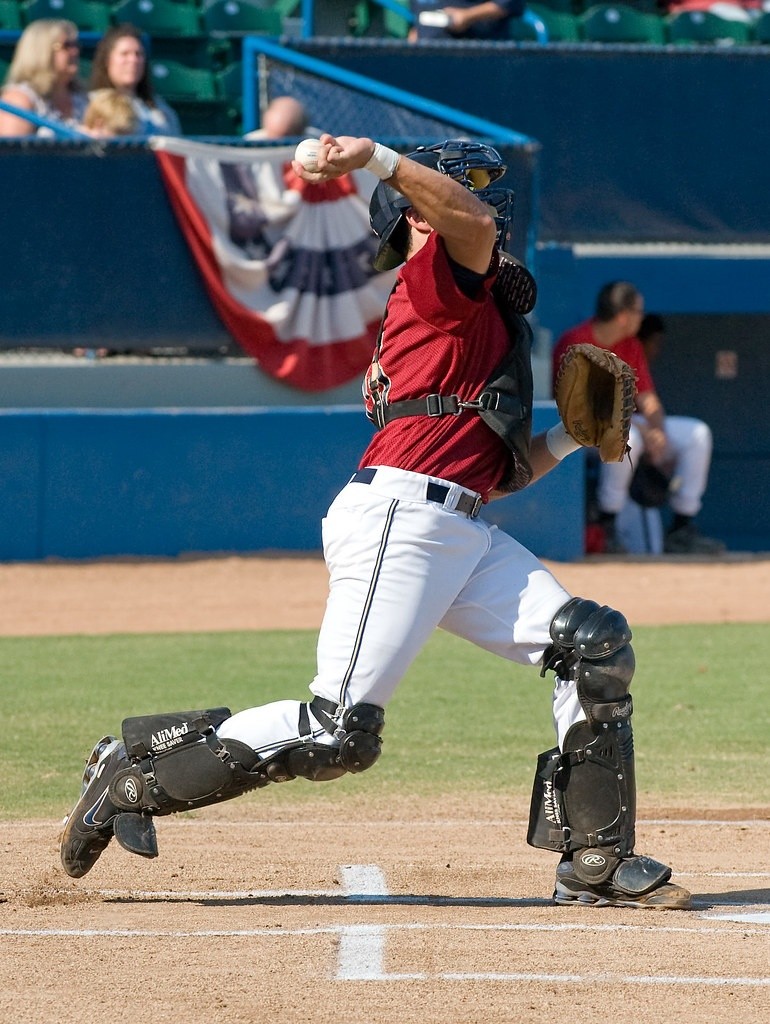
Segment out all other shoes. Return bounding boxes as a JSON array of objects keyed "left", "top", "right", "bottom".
[
  {"left": 667, "top": 529, "right": 729, "bottom": 552},
  {"left": 592, "top": 515, "right": 627, "bottom": 555}
]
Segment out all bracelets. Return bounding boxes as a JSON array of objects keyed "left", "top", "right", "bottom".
[{"left": 363, "top": 143, "right": 402, "bottom": 180}]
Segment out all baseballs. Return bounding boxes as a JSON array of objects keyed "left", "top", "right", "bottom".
[{"left": 294, "top": 138, "right": 325, "bottom": 174}]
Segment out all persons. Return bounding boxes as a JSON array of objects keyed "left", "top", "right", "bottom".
[
  {"left": 237, "top": 94, "right": 328, "bottom": 144},
  {"left": 404, "top": 0, "right": 529, "bottom": 46},
  {"left": 0, "top": 16, "right": 184, "bottom": 140},
  {"left": 550, "top": 278, "right": 730, "bottom": 557},
  {"left": 56, "top": 132, "right": 695, "bottom": 912}
]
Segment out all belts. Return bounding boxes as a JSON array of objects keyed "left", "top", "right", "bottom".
[{"left": 351, "top": 469, "right": 484, "bottom": 520}]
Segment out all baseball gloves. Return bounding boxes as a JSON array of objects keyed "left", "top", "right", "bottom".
[{"left": 552, "top": 342, "right": 638, "bottom": 465}]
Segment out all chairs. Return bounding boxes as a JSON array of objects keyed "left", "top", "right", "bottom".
[{"left": 0, "top": 0, "right": 770, "bottom": 135}]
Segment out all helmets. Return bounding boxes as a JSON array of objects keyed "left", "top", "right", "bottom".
[{"left": 367, "top": 140, "right": 492, "bottom": 273}]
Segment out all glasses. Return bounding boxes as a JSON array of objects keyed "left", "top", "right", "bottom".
[{"left": 52, "top": 39, "right": 79, "bottom": 51}]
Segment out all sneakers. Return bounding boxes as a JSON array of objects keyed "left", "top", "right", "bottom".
[
  {"left": 58, "top": 735, "right": 131, "bottom": 880},
  {"left": 555, "top": 851, "right": 695, "bottom": 910}
]
